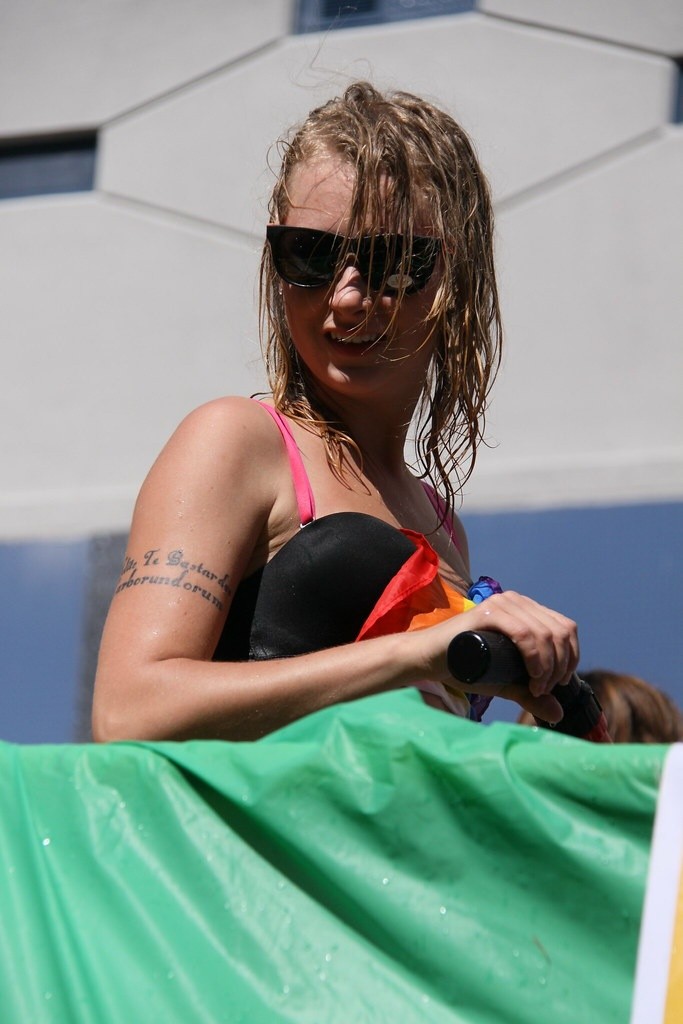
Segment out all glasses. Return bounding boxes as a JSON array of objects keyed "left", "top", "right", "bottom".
[{"left": 266, "top": 224, "right": 446, "bottom": 294}]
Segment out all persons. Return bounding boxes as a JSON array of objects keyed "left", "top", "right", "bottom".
[
  {"left": 89, "top": 80, "right": 583, "bottom": 746},
  {"left": 514, "top": 669, "right": 683, "bottom": 745}
]
